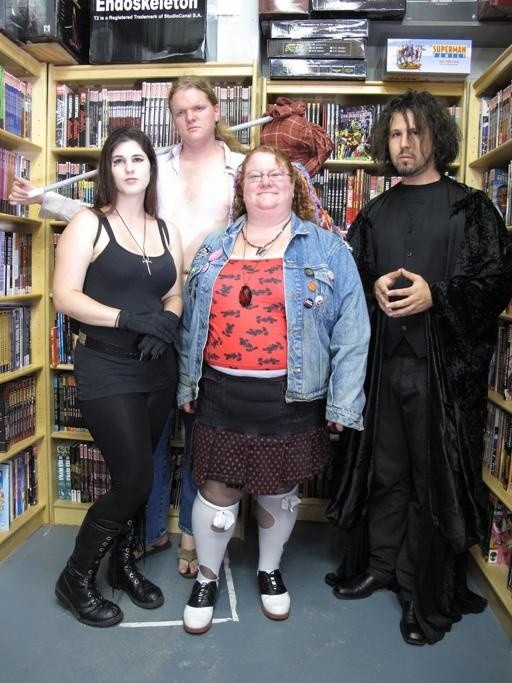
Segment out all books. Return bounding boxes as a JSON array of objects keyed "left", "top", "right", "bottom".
[
  {"left": 283, "top": 95, "right": 463, "bottom": 233},
  {"left": 0, "top": 66, "right": 36, "bottom": 530},
  {"left": 473, "top": 87, "right": 511, "bottom": 603},
  {"left": 49, "top": 312, "right": 184, "bottom": 511},
  {"left": 48, "top": 81, "right": 251, "bottom": 208}
]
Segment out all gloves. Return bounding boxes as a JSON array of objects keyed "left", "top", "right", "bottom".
[
  {"left": 139, "top": 311, "right": 179, "bottom": 358},
  {"left": 119, "top": 306, "right": 180, "bottom": 345}
]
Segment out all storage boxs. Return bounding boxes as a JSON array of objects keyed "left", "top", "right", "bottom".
[
  {"left": 87, "top": 0, "right": 209, "bottom": 63},
  {"left": 380, "top": 37, "right": 475, "bottom": 82}
]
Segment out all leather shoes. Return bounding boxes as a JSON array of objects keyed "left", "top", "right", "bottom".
[
  {"left": 256, "top": 566, "right": 291, "bottom": 619},
  {"left": 400, "top": 597, "right": 427, "bottom": 646},
  {"left": 182, "top": 574, "right": 220, "bottom": 633},
  {"left": 333, "top": 572, "right": 390, "bottom": 599}
]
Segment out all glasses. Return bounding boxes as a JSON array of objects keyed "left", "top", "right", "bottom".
[{"left": 241, "top": 169, "right": 294, "bottom": 183}]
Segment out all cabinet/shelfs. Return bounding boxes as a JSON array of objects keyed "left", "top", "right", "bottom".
[
  {"left": 0, "top": 34, "right": 50, "bottom": 560},
  {"left": 44, "top": 60, "right": 259, "bottom": 541},
  {"left": 465, "top": 43, "right": 510, "bottom": 628},
  {"left": 257, "top": 74, "right": 468, "bottom": 522}
]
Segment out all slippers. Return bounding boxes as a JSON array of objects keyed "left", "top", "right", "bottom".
[
  {"left": 178, "top": 536, "right": 199, "bottom": 580},
  {"left": 134, "top": 538, "right": 172, "bottom": 559}
]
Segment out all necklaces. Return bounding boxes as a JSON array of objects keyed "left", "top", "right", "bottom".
[
  {"left": 237, "top": 219, "right": 289, "bottom": 307},
  {"left": 111, "top": 202, "right": 159, "bottom": 277},
  {"left": 239, "top": 214, "right": 293, "bottom": 256}
]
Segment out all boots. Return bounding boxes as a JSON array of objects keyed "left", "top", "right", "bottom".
[
  {"left": 108, "top": 514, "right": 163, "bottom": 609},
  {"left": 55, "top": 509, "right": 124, "bottom": 627}
]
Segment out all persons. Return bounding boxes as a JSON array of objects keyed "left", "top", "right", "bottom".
[
  {"left": 6, "top": 73, "right": 250, "bottom": 579},
  {"left": 324, "top": 90, "right": 512, "bottom": 644},
  {"left": 172, "top": 134, "right": 372, "bottom": 634},
  {"left": 49, "top": 125, "right": 171, "bottom": 628}
]
